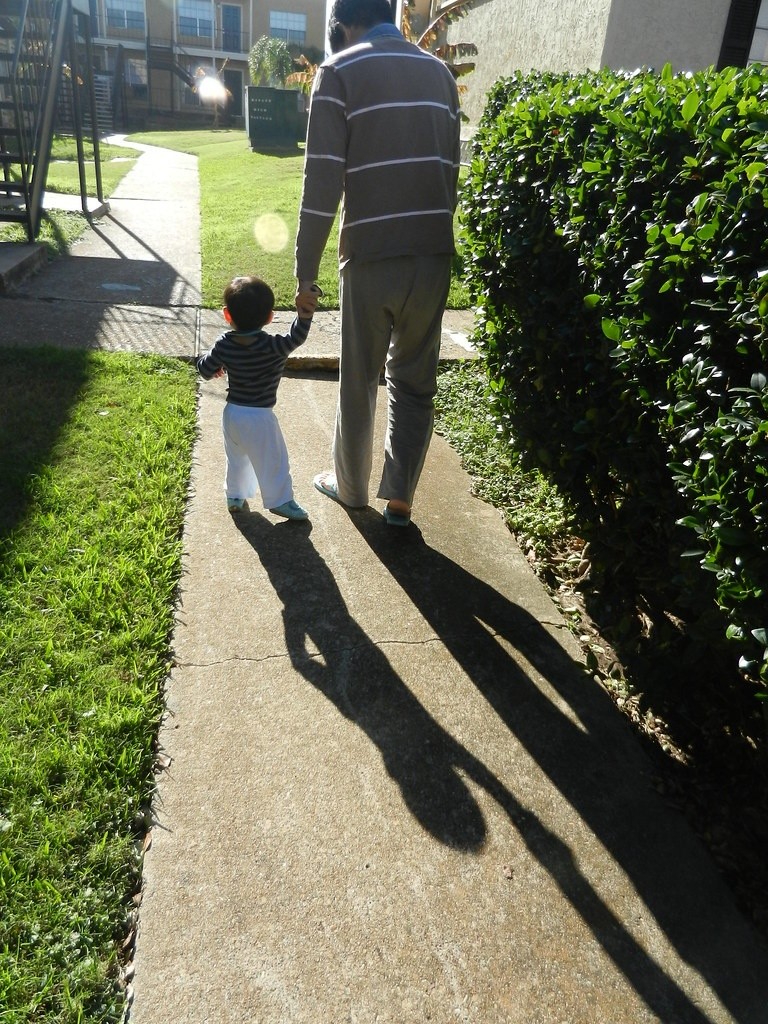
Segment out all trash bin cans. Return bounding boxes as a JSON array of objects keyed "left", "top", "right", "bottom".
[{"left": 245, "top": 86, "right": 301, "bottom": 154}]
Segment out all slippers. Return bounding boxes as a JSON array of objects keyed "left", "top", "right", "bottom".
[
  {"left": 313, "top": 473, "right": 341, "bottom": 501},
  {"left": 383, "top": 507, "right": 411, "bottom": 526}
]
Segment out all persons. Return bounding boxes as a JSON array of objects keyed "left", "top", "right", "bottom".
[
  {"left": 197, "top": 277, "right": 322, "bottom": 520},
  {"left": 293, "top": 0, "right": 461, "bottom": 526}
]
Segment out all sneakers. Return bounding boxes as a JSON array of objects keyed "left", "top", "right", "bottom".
[
  {"left": 269, "top": 500, "right": 309, "bottom": 520},
  {"left": 227, "top": 498, "right": 246, "bottom": 512}
]
